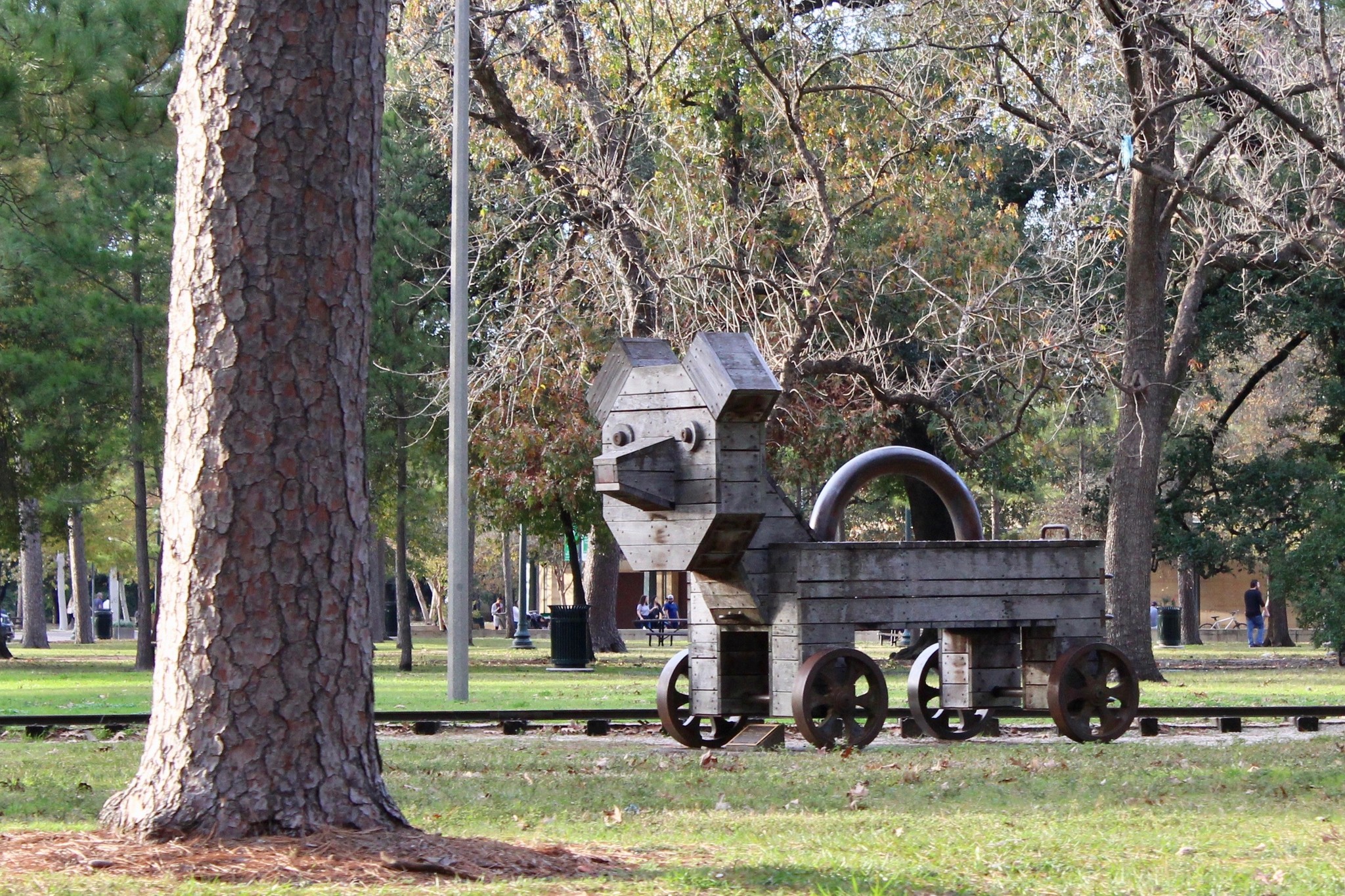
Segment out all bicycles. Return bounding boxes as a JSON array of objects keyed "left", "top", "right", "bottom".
[{"left": 1200, "top": 609, "right": 1247, "bottom": 629}]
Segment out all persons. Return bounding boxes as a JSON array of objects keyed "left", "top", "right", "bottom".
[
  {"left": 1149, "top": 601, "right": 1158, "bottom": 629},
  {"left": 636, "top": 594, "right": 680, "bottom": 629},
  {"left": 472, "top": 596, "right": 519, "bottom": 630},
  {"left": 67, "top": 597, "right": 75, "bottom": 624},
  {"left": 1244, "top": 579, "right": 1270, "bottom": 647},
  {"left": 103, "top": 596, "right": 110, "bottom": 610},
  {"left": 94, "top": 592, "right": 103, "bottom": 611}
]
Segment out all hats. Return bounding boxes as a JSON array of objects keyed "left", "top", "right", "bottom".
[
  {"left": 1152, "top": 601, "right": 1157, "bottom": 605},
  {"left": 668, "top": 594, "right": 674, "bottom": 598}
]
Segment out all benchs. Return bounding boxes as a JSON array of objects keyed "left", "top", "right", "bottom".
[
  {"left": 633, "top": 618, "right": 689, "bottom": 647},
  {"left": 11, "top": 617, "right": 24, "bottom": 630}
]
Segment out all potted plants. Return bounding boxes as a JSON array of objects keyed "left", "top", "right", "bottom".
[
  {"left": 113, "top": 619, "right": 134, "bottom": 639},
  {"left": 151, "top": 602, "right": 156, "bottom": 631},
  {"left": 471, "top": 610, "right": 485, "bottom": 629}
]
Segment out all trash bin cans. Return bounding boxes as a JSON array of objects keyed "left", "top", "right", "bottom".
[
  {"left": 548, "top": 604, "right": 592, "bottom": 668},
  {"left": 94, "top": 609, "right": 114, "bottom": 640},
  {"left": 1156, "top": 606, "right": 1182, "bottom": 646},
  {"left": 385, "top": 601, "right": 397, "bottom": 637}
]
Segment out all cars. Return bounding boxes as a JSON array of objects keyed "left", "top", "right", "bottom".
[{"left": 0, "top": 613, "right": 14, "bottom": 635}]
